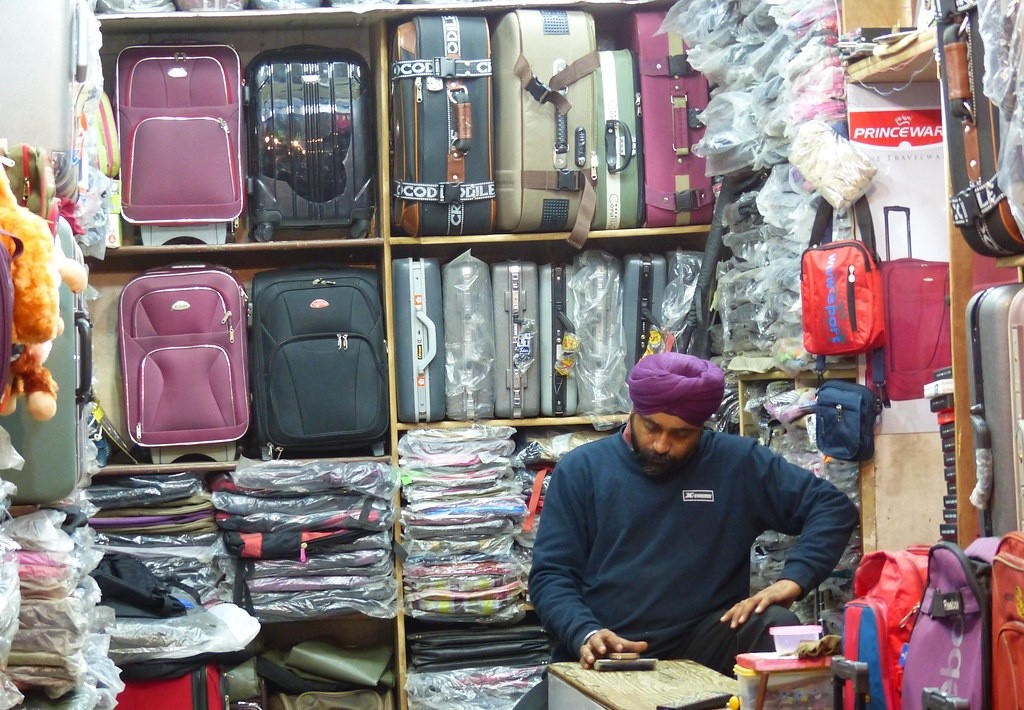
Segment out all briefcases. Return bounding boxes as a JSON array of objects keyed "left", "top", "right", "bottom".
[
  {"left": 394, "top": 13, "right": 712, "bottom": 237},
  {"left": 394, "top": 248, "right": 705, "bottom": 423},
  {"left": 878, "top": 201, "right": 949, "bottom": 400}
]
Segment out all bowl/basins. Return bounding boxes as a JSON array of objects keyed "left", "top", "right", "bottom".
[{"left": 768, "top": 625, "right": 822, "bottom": 652}]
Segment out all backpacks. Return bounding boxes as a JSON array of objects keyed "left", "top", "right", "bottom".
[
  {"left": 84, "top": 425, "right": 532, "bottom": 658},
  {"left": 843, "top": 529, "right": 1024, "bottom": 710}
]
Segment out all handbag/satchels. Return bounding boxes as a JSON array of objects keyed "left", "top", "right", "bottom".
[
  {"left": 800, "top": 241, "right": 887, "bottom": 354},
  {"left": 816, "top": 382, "right": 875, "bottom": 462},
  {"left": 92, "top": 555, "right": 202, "bottom": 618}
]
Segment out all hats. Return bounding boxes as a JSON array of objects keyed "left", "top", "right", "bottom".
[{"left": 628, "top": 351, "right": 726, "bottom": 425}]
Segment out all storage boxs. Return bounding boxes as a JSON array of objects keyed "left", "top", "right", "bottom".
[
  {"left": 944, "top": 451, "right": 955, "bottom": 467},
  {"left": 943, "top": 494, "right": 957, "bottom": 509},
  {"left": 938, "top": 408, "right": 954, "bottom": 425},
  {"left": 930, "top": 393, "right": 953, "bottom": 413},
  {"left": 940, "top": 523, "right": 957, "bottom": 543},
  {"left": 933, "top": 366, "right": 952, "bottom": 381},
  {"left": 947, "top": 479, "right": 956, "bottom": 495},
  {"left": 769, "top": 625, "right": 823, "bottom": 655},
  {"left": 943, "top": 508, "right": 957, "bottom": 523},
  {"left": 942, "top": 437, "right": 955, "bottom": 453},
  {"left": 923, "top": 379, "right": 954, "bottom": 400},
  {"left": 940, "top": 421, "right": 955, "bottom": 439},
  {"left": 944, "top": 465, "right": 955, "bottom": 481},
  {"left": 733, "top": 663, "right": 835, "bottom": 710}
]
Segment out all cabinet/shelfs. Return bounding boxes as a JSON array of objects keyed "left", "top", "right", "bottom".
[
  {"left": 84, "top": 1, "right": 721, "bottom": 710},
  {"left": 735, "top": 0, "right": 1024, "bottom": 553},
  {"left": 547, "top": 658, "right": 740, "bottom": 710}
]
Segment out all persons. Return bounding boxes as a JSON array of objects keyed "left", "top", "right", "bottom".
[{"left": 511, "top": 352, "right": 858, "bottom": 710}]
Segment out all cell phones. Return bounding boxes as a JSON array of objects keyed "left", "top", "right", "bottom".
[{"left": 609, "top": 652, "right": 640, "bottom": 660}]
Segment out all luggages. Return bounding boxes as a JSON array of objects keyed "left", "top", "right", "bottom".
[
  {"left": 241, "top": 46, "right": 370, "bottom": 237},
  {"left": 116, "top": 266, "right": 249, "bottom": 447},
  {"left": 965, "top": 284, "right": 1024, "bottom": 536},
  {"left": 113, "top": 38, "right": 246, "bottom": 246},
  {"left": 0, "top": 0, "right": 92, "bottom": 508},
  {"left": 252, "top": 252, "right": 389, "bottom": 462},
  {"left": 935, "top": 0, "right": 1024, "bottom": 257}
]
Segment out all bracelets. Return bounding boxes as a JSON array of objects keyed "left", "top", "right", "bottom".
[{"left": 581, "top": 629, "right": 599, "bottom": 646}]
